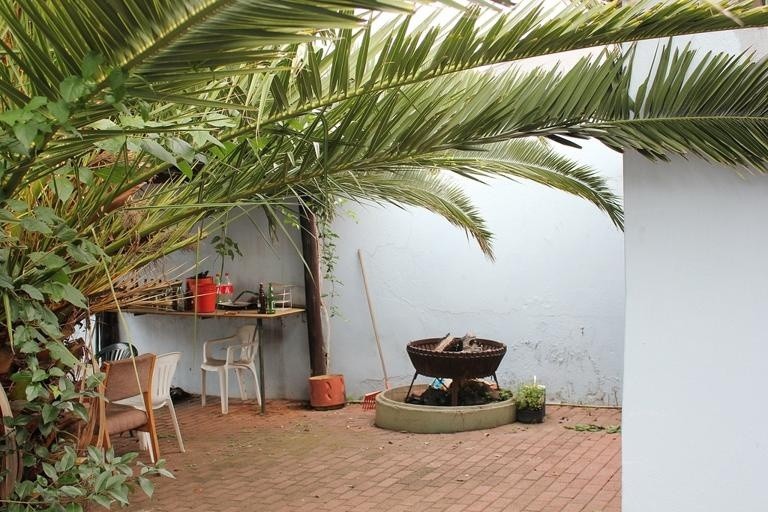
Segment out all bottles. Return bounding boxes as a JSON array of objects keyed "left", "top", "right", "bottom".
[
  {"left": 215, "top": 272, "right": 233, "bottom": 302},
  {"left": 177, "top": 281, "right": 193, "bottom": 310},
  {"left": 257, "top": 281, "right": 275, "bottom": 314}
]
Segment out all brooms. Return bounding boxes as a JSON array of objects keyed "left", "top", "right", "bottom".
[{"left": 358, "top": 250, "right": 389, "bottom": 410}]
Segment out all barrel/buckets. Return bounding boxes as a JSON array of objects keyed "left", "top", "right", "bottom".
[
  {"left": 185, "top": 276, "right": 213, "bottom": 292},
  {"left": 191, "top": 284, "right": 216, "bottom": 312}
]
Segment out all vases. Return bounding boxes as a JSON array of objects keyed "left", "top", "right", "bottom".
[{"left": 308, "top": 373, "right": 347, "bottom": 411}]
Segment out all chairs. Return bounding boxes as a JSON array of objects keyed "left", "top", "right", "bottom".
[{"left": 85, "top": 324, "right": 266, "bottom": 476}]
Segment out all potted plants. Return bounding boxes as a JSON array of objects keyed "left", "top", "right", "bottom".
[{"left": 516, "top": 383, "right": 546, "bottom": 422}]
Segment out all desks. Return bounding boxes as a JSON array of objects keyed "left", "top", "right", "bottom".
[{"left": 96, "top": 305, "right": 307, "bottom": 411}]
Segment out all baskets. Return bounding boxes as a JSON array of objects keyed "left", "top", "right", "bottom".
[{"left": 406, "top": 336, "right": 508, "bottom": 380}]
